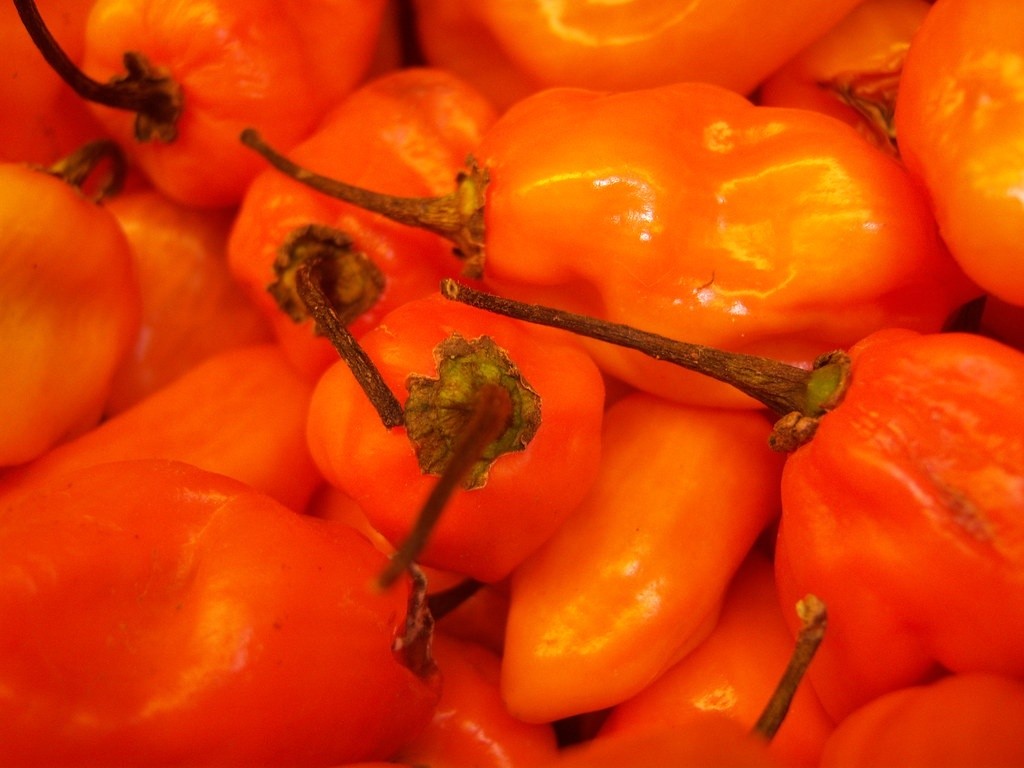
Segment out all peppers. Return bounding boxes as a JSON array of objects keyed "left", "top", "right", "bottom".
[{"left": 0, "top": 0, "right": 1024, "bottom": 767}]
